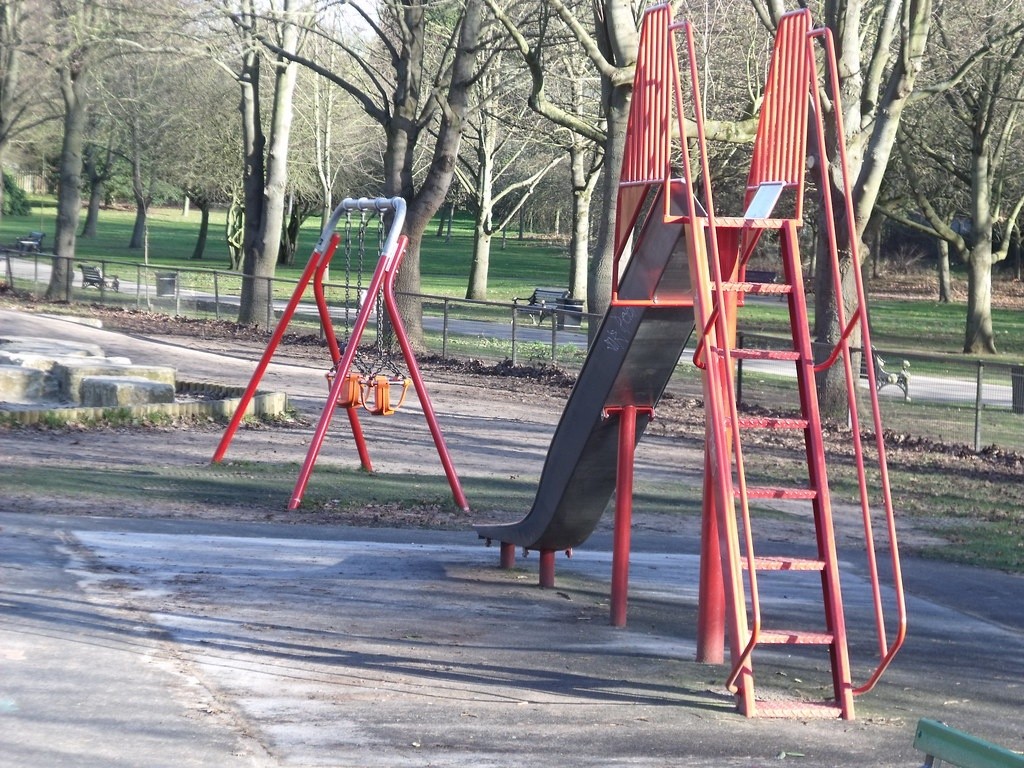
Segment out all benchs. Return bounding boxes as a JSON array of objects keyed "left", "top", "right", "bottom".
[
  {"left": 857, "top": 343, "right": 914, "bottom": 406},
  {"left": 78, "top": 264, "right": 120, "bottom": 295},
  {"left": 16, "top": 231, "right": 45, "bottom": 257},
  {"left": 511, "top": 286, "right": 570, "bottom": 328}
]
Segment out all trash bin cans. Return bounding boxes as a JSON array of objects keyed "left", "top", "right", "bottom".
[
  {"left": 1012, "top": 366, "right": 1023, "bottom": 415},
  {"left": 555, "top": 298, "right": 585, "bottom": 332},
  {"left": 155, "top": 272, "right": 176, "bottom": 299}
]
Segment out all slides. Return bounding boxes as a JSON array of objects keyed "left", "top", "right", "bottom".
[{"left": 469, "top": 178, "right": 785, "bottom": 551}]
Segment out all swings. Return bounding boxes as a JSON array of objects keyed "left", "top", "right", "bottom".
[
  {"left": 326, "top": 211, "right": 372, "bottom": 407},
  {"left": 358, "top": 213, "right": 412, "bottom": 416}
]
